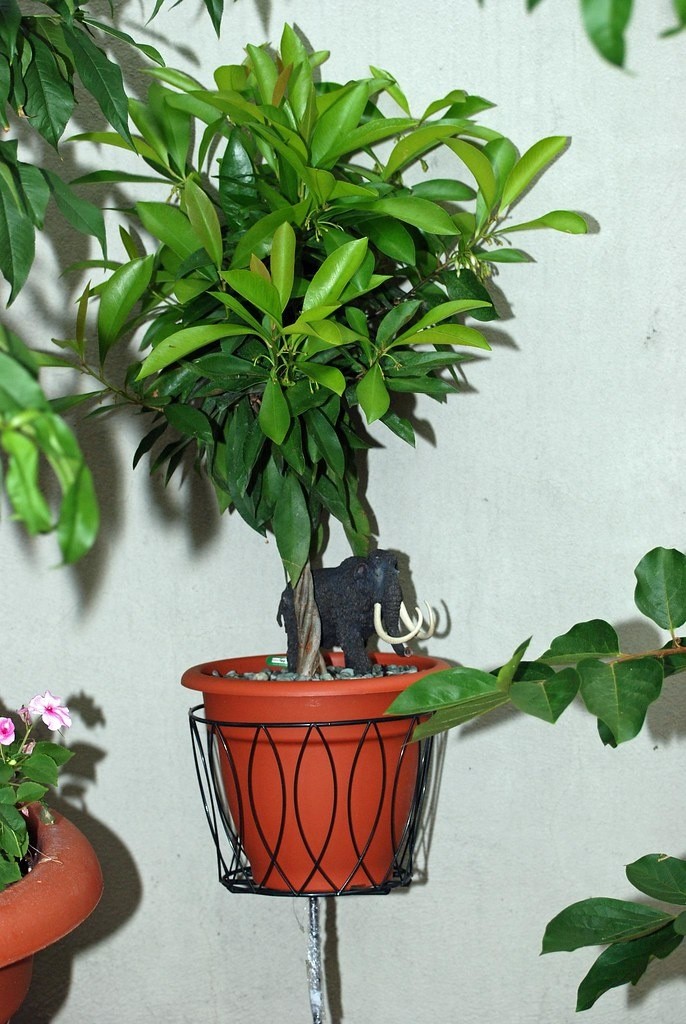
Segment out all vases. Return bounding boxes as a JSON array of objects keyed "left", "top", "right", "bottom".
[{"left": 0, "top": 805, "right": 107, "bottom": 1024}]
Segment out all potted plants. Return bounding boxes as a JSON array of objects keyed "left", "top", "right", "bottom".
[{"left": 47, "top": 24, "right": 601, "bottom": 891}]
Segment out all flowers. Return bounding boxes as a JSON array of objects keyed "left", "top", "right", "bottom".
[{"left": 0, "top": 692, "right": 76, "bottom": 894}]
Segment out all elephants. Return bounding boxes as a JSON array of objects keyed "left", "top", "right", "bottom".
[{"left": 276, "top": 549, "right": 435, "bottom": 676}]
